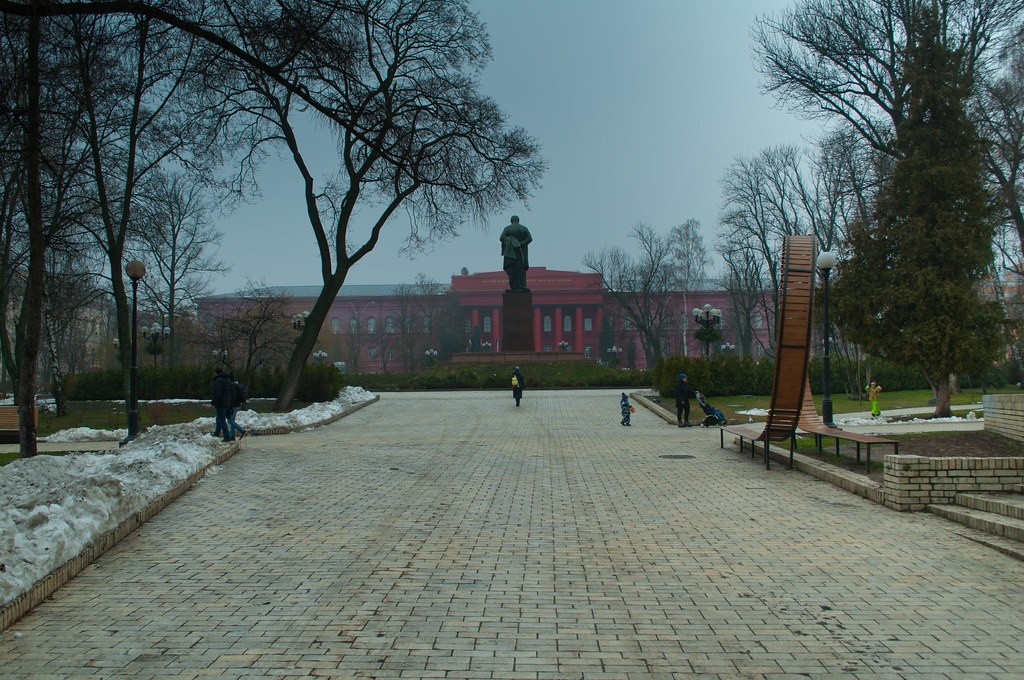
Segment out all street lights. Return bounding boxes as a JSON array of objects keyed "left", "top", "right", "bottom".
[
  {"left": 425, "top": 349, "right": 437, "bottom": 370},
  {"left": 607, "top": 346, "right": 623, "bottom": 371},
  {"left": 691, "top": 303, "right": 723, "bottom": 358},
  {"left": 141, "top": 324, "right": 173, "bottom": 397},
  {"left": 817, "top": 250, "right": 836, "bottom": 428},
  {"left": 347, "top": 301, "right": 375, "bottom": 377},
  {"left": 119, "top": 259, "right": 146, "bottom": 448}
]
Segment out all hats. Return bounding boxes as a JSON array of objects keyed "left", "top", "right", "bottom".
[
  {"left": 622, "top": 393, "right": 628, "bottom": 401},
  {"left": 678, "top": 374, "right": 686, "bottom": 379}
]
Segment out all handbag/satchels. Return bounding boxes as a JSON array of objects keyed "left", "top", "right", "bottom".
[{"left": 512, "top": 374, "right": 518, "bottom": 387}]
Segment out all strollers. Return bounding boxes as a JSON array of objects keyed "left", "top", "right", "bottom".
[{"left": 696, "top": 389, "right": 727, "bottom": 429}]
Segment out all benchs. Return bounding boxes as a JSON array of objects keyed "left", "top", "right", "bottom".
[
  {"left": 813, "top": 426, "right": 900, "bottom": 475},
  {"left": 719, "top": 425, "right": 796, "bottom": 472},
  {"left": 0, "top": 405, "right": 39, "bottom": 440}
]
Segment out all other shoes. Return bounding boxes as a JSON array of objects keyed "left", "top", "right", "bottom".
[
  {"left": 230, "top": 437, "right": 236, "bottom": 441},
  {"left": 222, "top": 438, "right": 230, "bottom": 442},
  {"left": 239, "top": 431, "right": 247, "bottom": 440}
]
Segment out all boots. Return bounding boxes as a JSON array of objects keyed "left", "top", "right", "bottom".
[
  {"left": 678, "top": 421, "right": 684, "bottom": 427},
  {"left": 685, "top": 421, "right": 692, "bottom": 426}
]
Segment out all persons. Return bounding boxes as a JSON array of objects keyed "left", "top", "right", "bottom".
[
  {"left": 211, "top": 366, "right": 247, "bottom": 443},
  {"left": 620, "top": 392, "right": 632, "bottom": 426},
  {"left": 499, "top": 215, "right": 533, "bottom": 290},
  {"left": 675, "top": 373, "right": 694, "bottom": 427},
  {"left": 511, "top": 366, "right": 524, "bottom": 407},
  {"left": 865, "top": 382, "right": 882, "bottom": 417}
]
